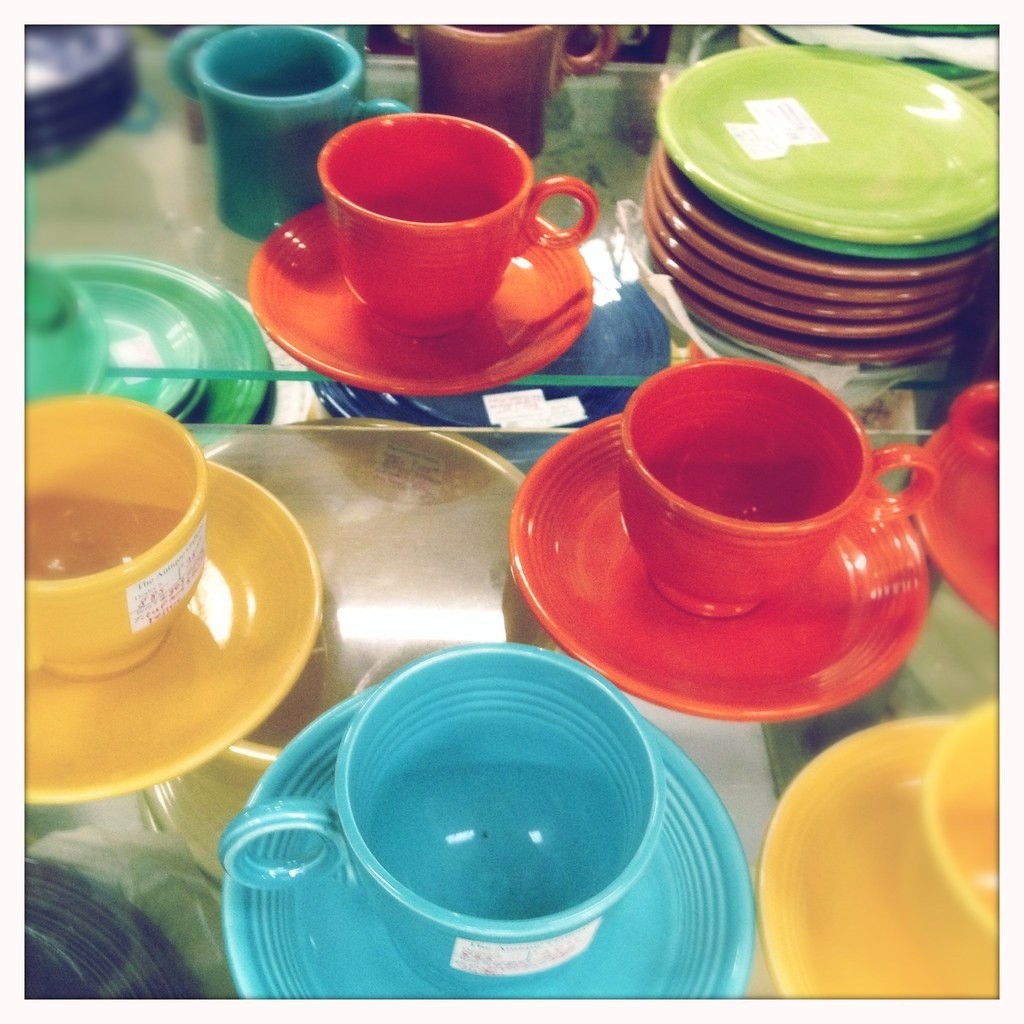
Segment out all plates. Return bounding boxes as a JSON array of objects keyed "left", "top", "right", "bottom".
[
  {"left": 74, "top": 279, "right": 201, "bottom": 415},
  {"left": 25, "top": 858, "right": 207, "bottom": 1000},
  {"left": 309, "top": 272, "right": 671, "bottom": 465},
  {"left": 759, "top": 715, "right": 999, "bottom": 999},
  {"left": 146, "top": 417, "right": 524, "bottom": 883},
  {"left": 911, "top": 423, "right": 999, "bottom": 624},
  {"left": 508, "top": 411, "right": 931, "bottom": 722},
  {"left": 25, "top": 24, "right": 138, "bottom": 153},
  {"left": 221, "top": 682, "right": 756, "bottom": 999},
  {"left": 250, "top": 201, "right": 596, "bottom": 396},
  {"left": 645, "top": 48, "right": 1002, "bottom": 375},
  {"left": 25, "top": 253, "right": 273, "bottom": 448},
  {"left": 26, "top": 460, "right": 325, "bottom": 804},
  {"left": 174, "top": 355, "right": 208, "bottom": 423}
]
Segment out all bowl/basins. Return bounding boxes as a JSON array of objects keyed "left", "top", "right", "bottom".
[{"left": 25, "top": 393, "right": 208, "bottom": 675}]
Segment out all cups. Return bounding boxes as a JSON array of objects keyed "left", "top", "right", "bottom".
[
  {"left": 167, "top": 25, "right": 368, "bottom": 111},
  {"left": 418, "top": 25, "right": 616, "bottom": 159},
  {"left": 619, "top": 357, "right": 939, "bottom": 619},
  {"left": 217, "top": 642, "right": 666, "bottom": 990},
  {"left": 25, "top": 263, "right": 108, "bottom": 395},
  {"left": 194, "top": 25, "right": 416, "bottom": 243},
  {"left": 925, "top": 701, "right": 999, "bottom": 934},
  {"left": 953, "top": 386, "right": 999, "bottom": 521},
  {"left": 316, "top": 112, "right": 599, "bottom": 333}
]
